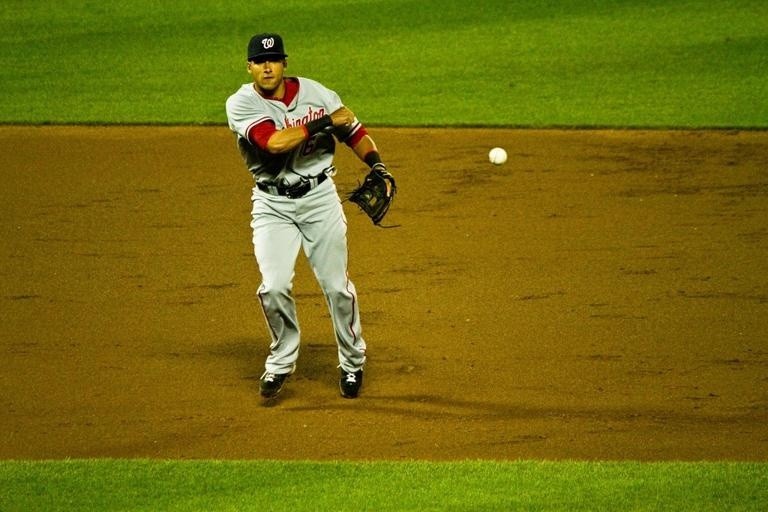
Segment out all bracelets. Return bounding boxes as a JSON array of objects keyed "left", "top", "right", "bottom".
[
  {"left": 301, "top": 114, "right": 333, "bottom": 140},
  {"left": 363, "top": 150, "right": 386, "bottom": 171}
]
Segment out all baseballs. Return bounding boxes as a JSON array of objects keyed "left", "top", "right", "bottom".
[{"left": 488, "top": 147, "right": 507, "bottom": 166}]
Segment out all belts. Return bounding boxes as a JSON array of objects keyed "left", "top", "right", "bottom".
[{"left": 256, "top": 164, "right": 335, "bottom": 199}]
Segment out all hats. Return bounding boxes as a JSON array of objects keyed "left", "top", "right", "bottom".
[{"left": 248, "top": 34, "right": 288, "bottom": 61}]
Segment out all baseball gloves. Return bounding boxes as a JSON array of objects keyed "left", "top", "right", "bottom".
[{"left": 342, "top": 163, "right": 400, "bottom": 228}]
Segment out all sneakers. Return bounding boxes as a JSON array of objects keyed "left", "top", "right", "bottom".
[
  {"left": 338, "top": 367, "right": 363, "bottom": 399},
  {"left": 259, "top": 372, "right": 290, "bottom": 399}
]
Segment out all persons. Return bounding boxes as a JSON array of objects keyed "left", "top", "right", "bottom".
[{"left": 225, "top": 34, "right": 395, "bottom": 398}]
